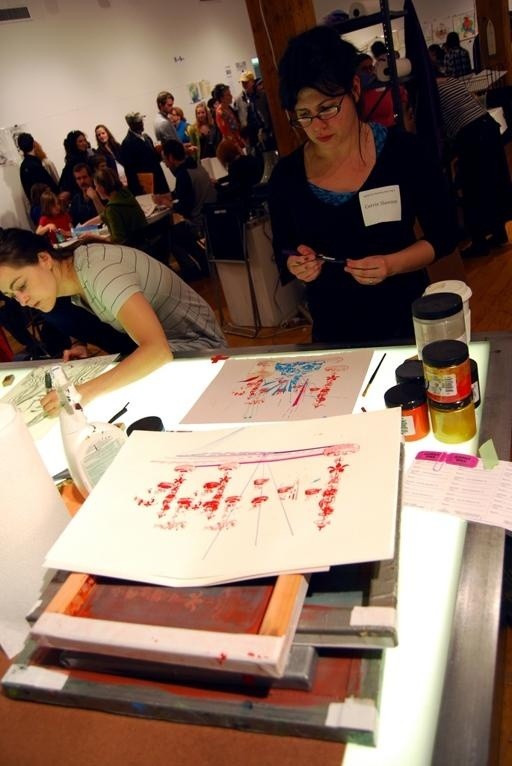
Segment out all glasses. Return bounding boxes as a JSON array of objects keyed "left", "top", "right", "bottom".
[{"left": 289, "top": 95, "right": 344, "bottom": 128}]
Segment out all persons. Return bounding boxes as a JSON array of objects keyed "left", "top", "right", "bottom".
[
  {"left": 18, "top": 72, "right": 278, "bottom": 260},
  {"left": 265, "top": 29, "right": 462, "bottom": 345},
  {"left": 1, "top": 226, "right": 229, "bottom": 415},
  {"left": 360, "top": 31, "right": 511, "bottom": 259}
]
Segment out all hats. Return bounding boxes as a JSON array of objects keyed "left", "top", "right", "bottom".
[
  {"left": 238, "top": 71, "right": 254, "bottom": 83},
  {"left": 124, "top": 110, "right": 146, "bottom": 123}
]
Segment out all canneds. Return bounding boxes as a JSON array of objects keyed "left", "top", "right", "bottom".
[{"left": 384, "top": 292, "right": 481, "bottom": 443}]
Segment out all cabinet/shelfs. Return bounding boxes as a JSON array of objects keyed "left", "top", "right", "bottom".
[{"left": 317, "top": 0, "right": 425, "bottom": 135}]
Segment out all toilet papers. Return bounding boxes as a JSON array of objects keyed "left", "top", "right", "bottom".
[
  {"left": 375, "top": 58, "right": 413, "bottom": 82},
  {"left": 349, "top": 0, "right": 405, "bottom": 19}
]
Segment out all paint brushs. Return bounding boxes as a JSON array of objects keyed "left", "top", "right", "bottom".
[{"left": 361, "top": 353, "right": 386, "bottom": 399}]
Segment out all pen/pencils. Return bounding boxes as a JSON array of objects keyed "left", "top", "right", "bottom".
[
  {"left": 108, "top": 401, "right": 130, "bottom": 424},
  {"left": 44, "top": 371, "right": 53, "bottom": 392},
  {"left": 315, "top": 251, "right": 348, "bottom": 266}
]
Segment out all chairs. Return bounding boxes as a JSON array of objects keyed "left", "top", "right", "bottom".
[{"left": 0, "top": 116, "right": 269, "bottom": 348}]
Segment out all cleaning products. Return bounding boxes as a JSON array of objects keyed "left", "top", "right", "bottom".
[{"left": 55, "top": 382, "right": 129, "bottom": 501}]
